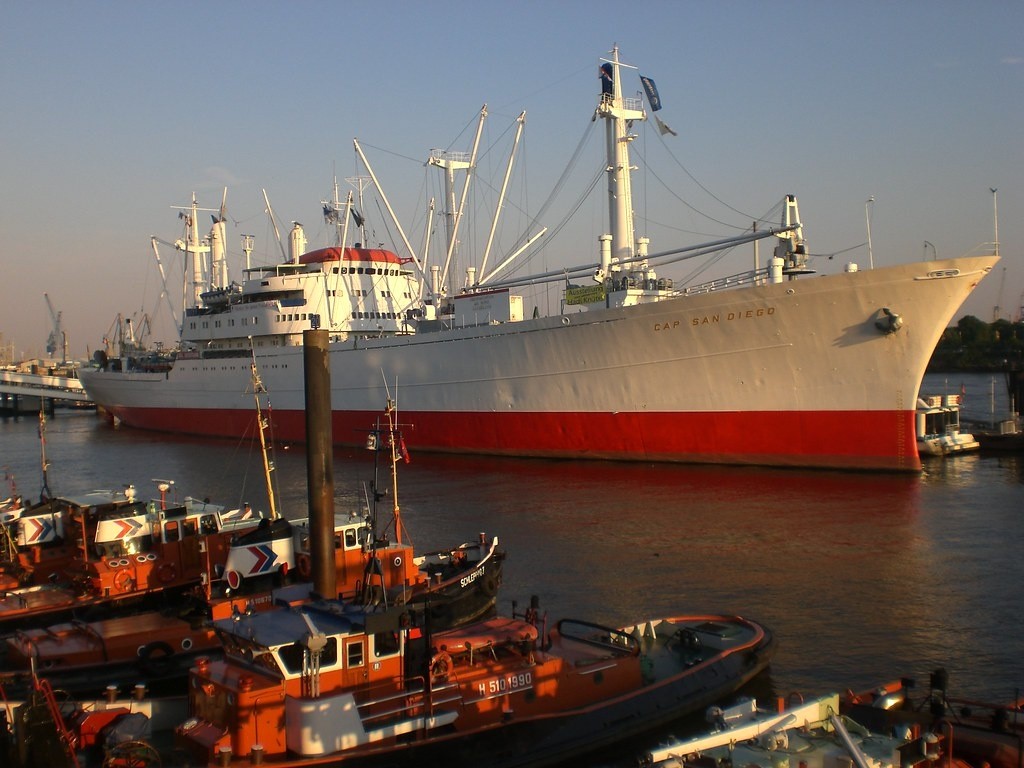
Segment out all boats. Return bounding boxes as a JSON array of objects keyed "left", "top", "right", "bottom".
[
  {"left": 959, "top": 376, "right": 1024, "bottom": 451},
  {"left": 0, "top": 315, "right": 1024, "bottom": 768},
  {"left": 914, "top": 377, "right": 980, "bottom": 456},
  {"left": 74, "top": 45, "right": 1001, "bottom": 472}
]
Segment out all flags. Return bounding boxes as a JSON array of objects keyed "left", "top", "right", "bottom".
[
  {"left": 397, "top": 438, "right": 411, "bottom": 464},
  {"left": 639, "top": 74, "right": 662, "bottom": 112},
  {"left": 655, "top": 115, "right": 678, "bottom": 137}
]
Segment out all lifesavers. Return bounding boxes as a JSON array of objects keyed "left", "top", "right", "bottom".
[
  {"left": 113, "top": 569, "right": 136, "bottom": 593},
  {"left": 428, "top": 652, "right": 453, "bottom": 684},
  {"left": 156, "top": 560, "right": 177, "bottom": 583},
  {"left": 297, "top": 555, "right": 311, "bottom": 577}
]
